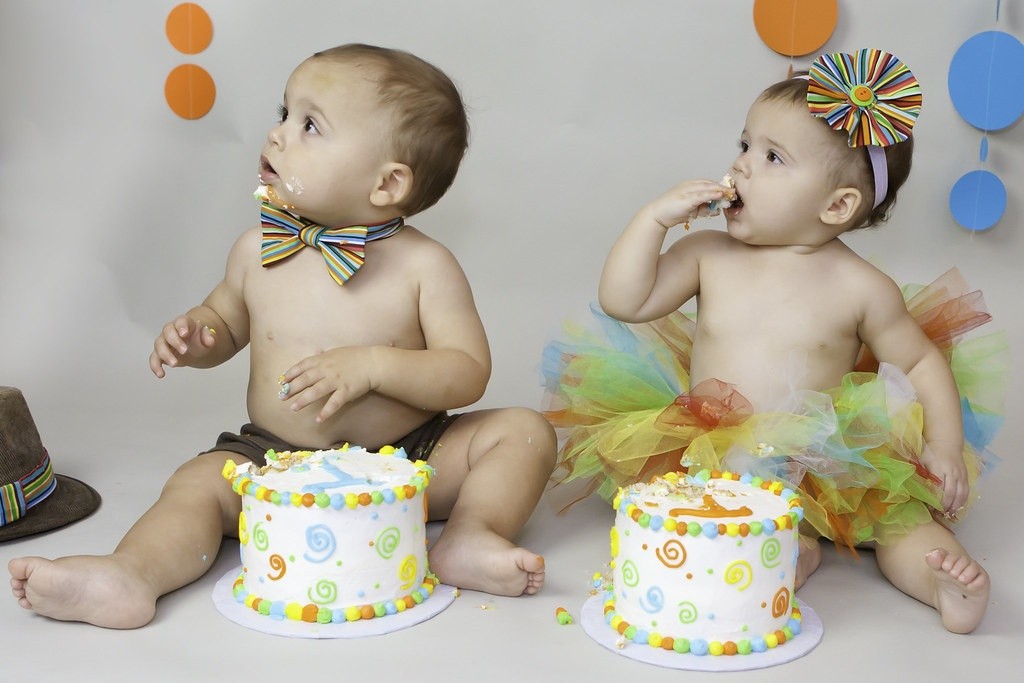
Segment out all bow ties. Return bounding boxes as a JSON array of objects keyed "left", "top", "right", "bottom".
[{"left": 260, "top": 202, "right": 405, "bottom": 286}]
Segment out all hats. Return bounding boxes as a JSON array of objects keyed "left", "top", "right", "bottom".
[{"left": 0, "top": 385, "right": 103, "bottom": 543}]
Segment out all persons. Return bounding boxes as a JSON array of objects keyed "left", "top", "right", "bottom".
[
  {"left": 533, "top": 49, "right": 990, "bottom": 635},
  {"left": 9, "top": 44, "right": 557, "bottom": 630}
]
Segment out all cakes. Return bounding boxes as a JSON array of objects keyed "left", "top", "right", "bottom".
[
  {"left": 221, "top": 443, "right": 437, "bottom": 622},
  {"left": 710, "top": 174, "right": 734, "bottom": 211},
  {"left": 604, "top": 469, "right": 803, "bottom": 656}
]
